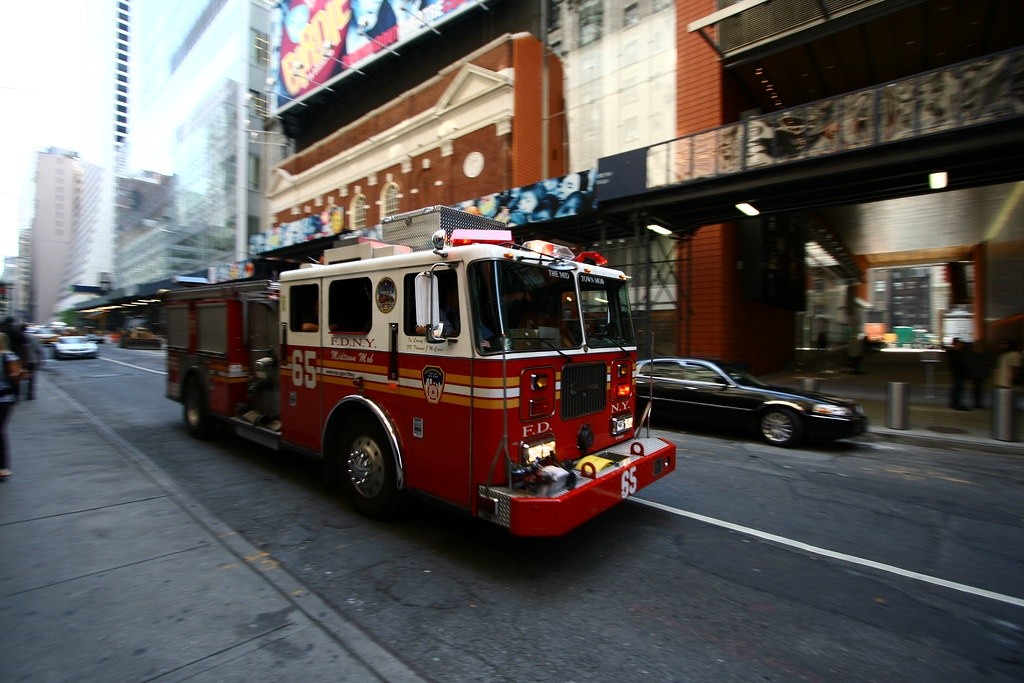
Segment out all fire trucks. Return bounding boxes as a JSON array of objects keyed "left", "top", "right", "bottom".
[{"left": 167, "top": 209, "right": 676, "bottom": 539}]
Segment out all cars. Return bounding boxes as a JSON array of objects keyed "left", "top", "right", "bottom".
[
  {"left": 50, "top": 336, "right": 98, "bottom": 358},
  {"left": 635, "top": 359, "right": 870, "bottom": 451}
]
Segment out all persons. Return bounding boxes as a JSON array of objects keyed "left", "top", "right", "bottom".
[
  {"left": 0, "top": 317, "right": 43, "bottom": 477},
  {"left": 301, "top": 300, "right": 339, "bottom": 331},
  {"left": 948, "top": 337, "right": 1024, "bottom": 412},
  {"left": 455, "top": 171, "right": 590, "bottom": 228},
  {"left": 416, "top": 275, "right": 495, "bottom": 347}
]
